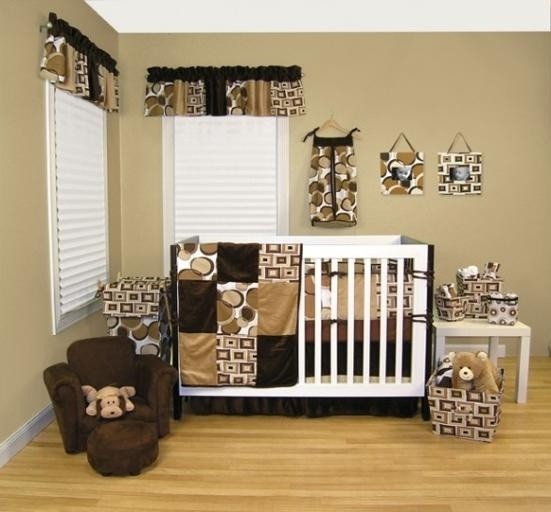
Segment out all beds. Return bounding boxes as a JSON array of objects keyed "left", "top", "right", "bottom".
[{"left": 167, "top": 231, "right": 437, "bottom": 423}]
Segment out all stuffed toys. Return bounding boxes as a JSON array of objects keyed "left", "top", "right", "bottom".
[
  {"left": 82, "top": 382, "right": 138, "bottom": 422},
  {"left": 449, "top": 349, "right": 502, "bottom": 399}
]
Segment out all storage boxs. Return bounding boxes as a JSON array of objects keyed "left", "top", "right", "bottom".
[{"left": 424, "top": 359, "right": 507, "bottom": 446}]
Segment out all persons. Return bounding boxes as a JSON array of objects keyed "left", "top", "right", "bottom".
[
  {"left": 391, "top": 166, "right": 413, "bottom": 181},
  {"left": 448, "top": 165, "right": 471, "bottom": 183}
]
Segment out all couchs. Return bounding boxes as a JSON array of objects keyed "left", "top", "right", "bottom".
[{"left": 41, "top": 336, "right": 180, "bottom": 478}]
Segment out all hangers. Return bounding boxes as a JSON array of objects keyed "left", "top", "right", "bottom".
[{"left": 301, "top": 109, "right": 366, "bottom": 145}]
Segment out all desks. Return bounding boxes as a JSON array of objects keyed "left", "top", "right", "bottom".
[{"left": 432, "top": 316, "right": 535, "bottom": 408}]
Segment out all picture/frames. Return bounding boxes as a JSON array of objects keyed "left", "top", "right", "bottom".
[
  {"left": 435, "top": 151, "right": 484, "bottom": 197},
  {"left": 378, "top": 151, "right": 424, "bottom": 197}
]
